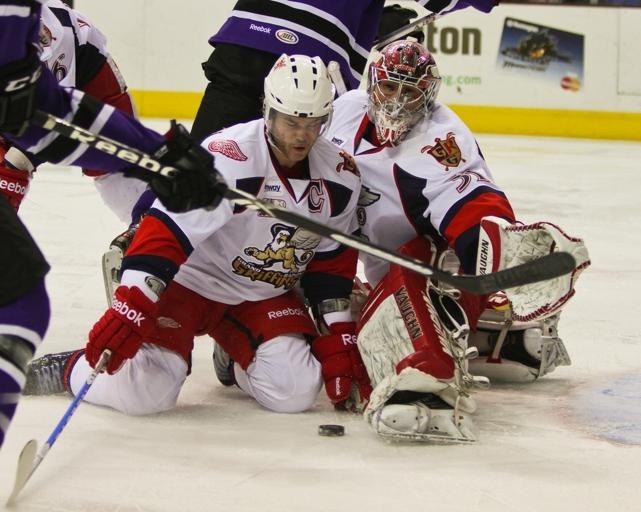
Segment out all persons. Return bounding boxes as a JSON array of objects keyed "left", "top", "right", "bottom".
[
  {"left": 21, "top": 53, "right": 375, "bottom": 417},
  {"left": 1, "top": 1, "right": 227, "bottom": 454},
  {"left": 319, "top": 39, "right": 591, "bottom": 444},
  {"left": 103, "top": 1, "right": 499, "bottom": 306}
]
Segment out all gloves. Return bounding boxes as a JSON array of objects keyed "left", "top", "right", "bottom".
[
  {"left": 85, "top": 285, "right": 160, "bottom": 374},
  {"left": 311, "top": 321, "right": 371, "bottom": 414},
  {"left": 373, "top": 4, "right": 426, "bottom": 46},
  {"left": 123, "top": 120, "right": 225, "bottom": 212}
]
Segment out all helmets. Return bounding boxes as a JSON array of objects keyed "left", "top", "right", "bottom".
[
  {"left": 264, "top": 52, "right": 333, "bottom": 147},
  {"left": 368, "top": 40, "right": 442, "bottom": 143}
]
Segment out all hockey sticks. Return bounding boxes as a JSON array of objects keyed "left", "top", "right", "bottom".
[
  {"left": 6, "top": 349, "right": 111, "bottom": 505},
  {"left": 32, "top": 111, "right": 574, "bottom": 294}
]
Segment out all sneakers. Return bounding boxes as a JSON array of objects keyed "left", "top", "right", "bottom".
[
  {"left": 479, "top": 327, "right": 546, "bottom": 368},
  {"left": 381, "top": 394, "right": 469, "bottom": 428},
  {"left": 23, "top": 350, "right": 81, "bottom": 395},
  {"left": 213, "top": 343, "right": 235, "bottom": 386}
]
